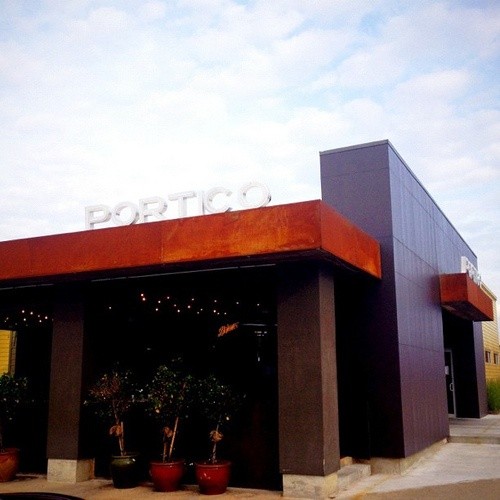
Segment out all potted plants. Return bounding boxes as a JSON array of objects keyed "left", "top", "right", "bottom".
[
  {"left": 195, "top": 375, "right": 240, "bottom": 495},
  {"left": 0, "top": 373, "right": 33, "bottom": 482},
  {"left": 141, "top": 357, "right": 199, "bottom": 491},
  {"left": 85, "top": 362, "right": 138, "bottom": 489}
]
